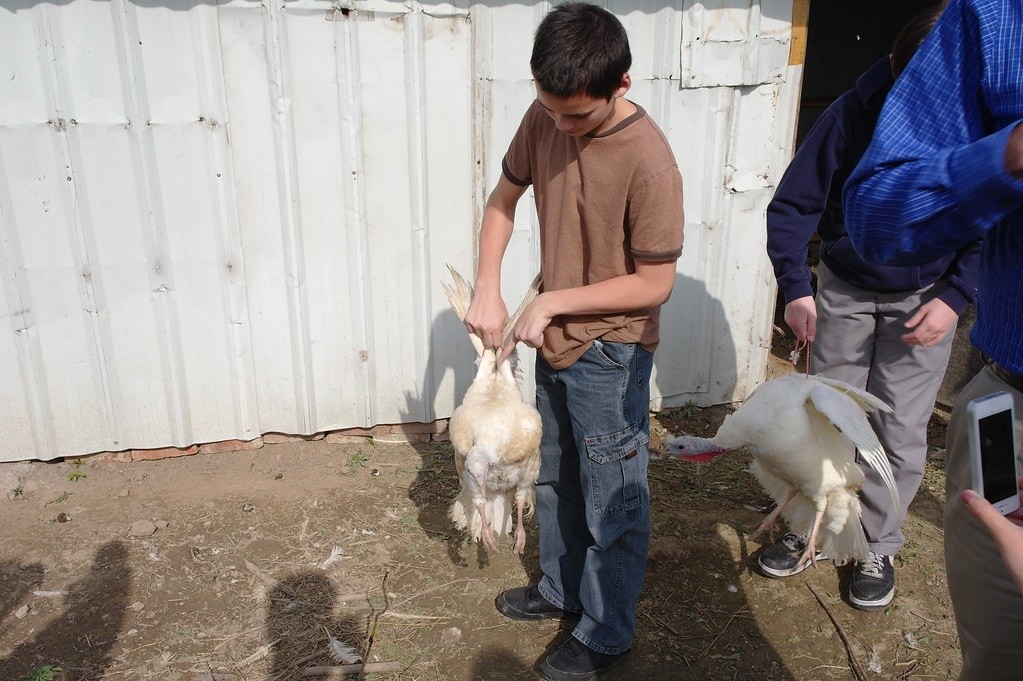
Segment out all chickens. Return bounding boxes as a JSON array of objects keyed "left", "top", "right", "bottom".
[
  {"left": 437, "top": 260, "right": 546, "bottom": 555},
  {"left": 656, "top": 368, "right": 903, "bottom": 575}
]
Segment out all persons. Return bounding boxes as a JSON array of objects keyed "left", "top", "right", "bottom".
[
  {"left": 843, "top": 1, "right": 1023, "bottom": 681},
  {"left": 463, "top": 4, "right": 685, "bottom": 678},
  {"left": 961, "top": 479, "right": 1023, "bottom": 593},
  {"left": 756, "top": 2, "right": 981, "bottom": 608}
]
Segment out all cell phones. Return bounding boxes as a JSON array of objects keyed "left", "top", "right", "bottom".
[{"left": 966, "top": 391, "right": 1020, "bottom": 518}]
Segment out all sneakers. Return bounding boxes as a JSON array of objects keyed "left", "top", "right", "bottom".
[
  {"left": 757, "top": 531, "right": 830, "bottom": 578},
  {"left": 539, "top": 635, "right": 631, "bottom": 681},
  {"left": 848, "top": 550, "right": 896, "bottom": 611},
  {"left": 500, "top": 582, "right": 582, "bottom": 620}
]
